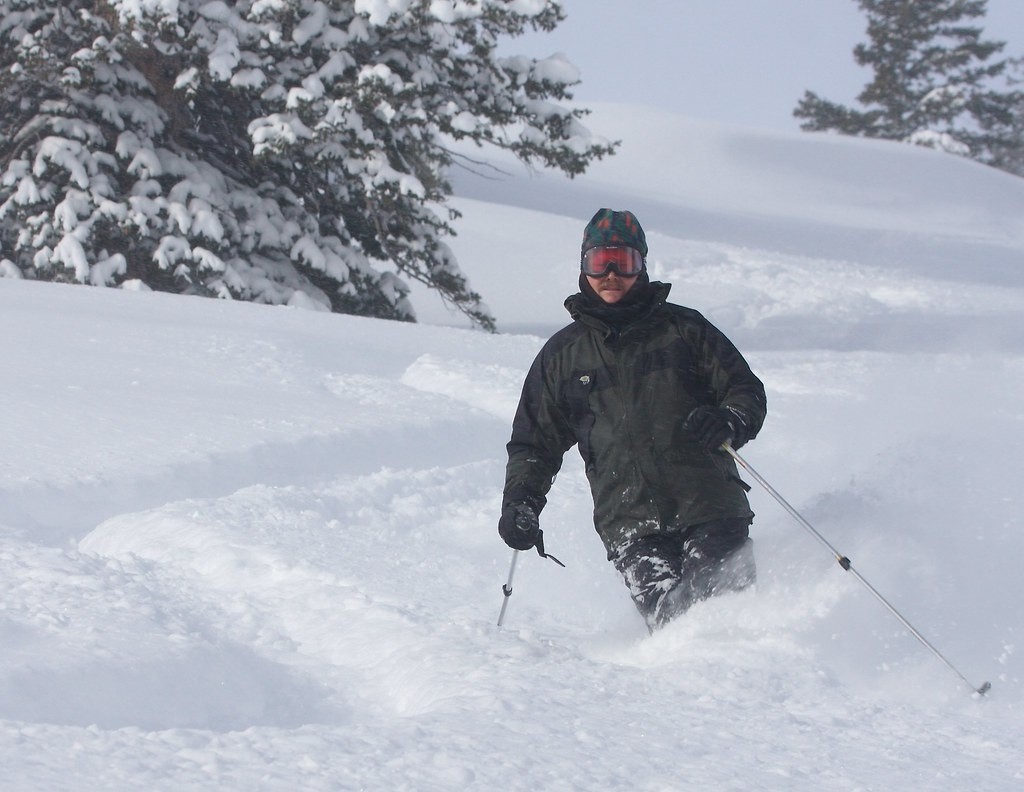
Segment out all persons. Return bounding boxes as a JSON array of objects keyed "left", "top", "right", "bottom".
[{"left": 500, "top": 210, "right": 768, "bottom": 632}]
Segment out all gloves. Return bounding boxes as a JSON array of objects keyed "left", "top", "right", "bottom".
[
  {"left": 680, "top": 405, "right": 750, "bottom": 454},
  {"left": 498, "top": 486, "right": 547, "bottom": 550}
]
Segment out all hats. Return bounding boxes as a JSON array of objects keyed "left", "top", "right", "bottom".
[{"left": 580, "top": 208, "right": 648, "bottom": 259}]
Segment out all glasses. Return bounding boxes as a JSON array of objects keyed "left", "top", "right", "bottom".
[{"left": 582, "top": 245, "right": 644, "bottom": 277}]
